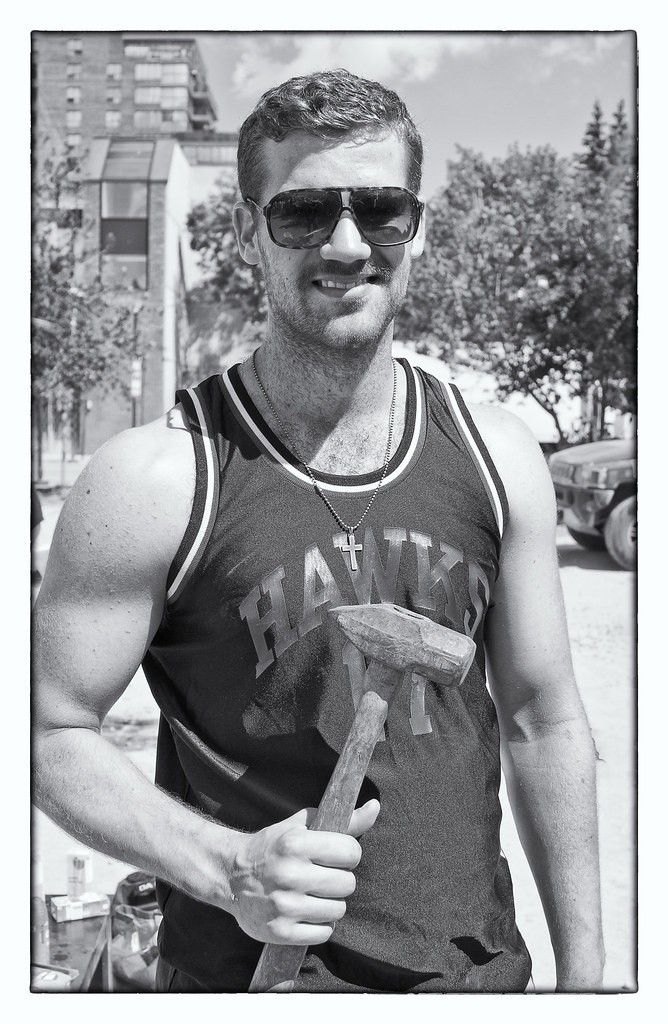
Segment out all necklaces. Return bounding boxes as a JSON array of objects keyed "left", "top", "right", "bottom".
[{"left": 251, "top": 346, "right": 397, "bottom": 570}]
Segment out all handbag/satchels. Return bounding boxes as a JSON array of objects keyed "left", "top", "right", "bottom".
[{"left": 109, "top": 871, "right": 164, "bottom": 991}]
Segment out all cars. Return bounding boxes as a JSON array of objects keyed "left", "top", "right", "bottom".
[{"left": 549, "top": 437, "right": 637, "bottom": 570}]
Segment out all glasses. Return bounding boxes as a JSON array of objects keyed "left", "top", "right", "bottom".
[{"left": 246, "top": 186, "right": 426, "bottom": 252}]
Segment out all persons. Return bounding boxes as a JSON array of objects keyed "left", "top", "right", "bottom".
[{"left": 31, "top": 72, "right": 603, "bottom": 992}]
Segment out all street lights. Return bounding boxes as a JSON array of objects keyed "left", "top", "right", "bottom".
[{"left": 123, "top": 282, "right": 146, "bottom": 428}]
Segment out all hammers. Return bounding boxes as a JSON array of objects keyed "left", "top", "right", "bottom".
[{"left": 249, "top": 602, "right": 477, "bottom": 993}]
